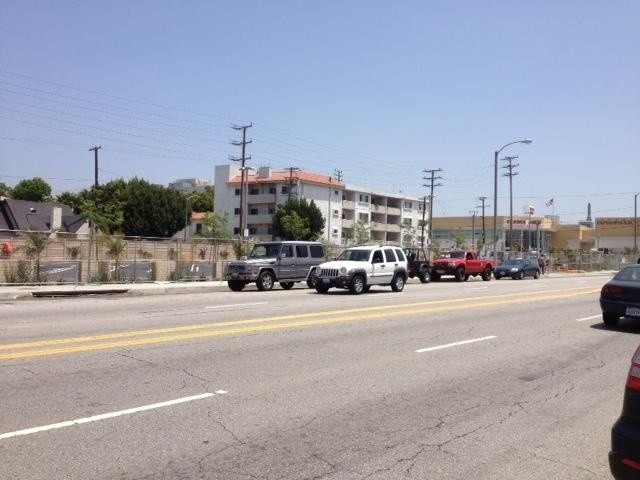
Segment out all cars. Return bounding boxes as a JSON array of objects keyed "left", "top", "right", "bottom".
[
  {"left": 403, "top": 247, "right": 432, "bottom": 284},
  {"left": 599, "top": 263, "right": 640, "bottom": 327},
  {"left": 493, "top": 257, "right": 542, "bottom": 281},
  {"left": 608, "top": 346, "right": 640, "bottom": 480}
]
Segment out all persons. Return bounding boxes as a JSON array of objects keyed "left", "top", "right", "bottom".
[{"left": 404, "top": 248, "right": 416, "bottom": 261}]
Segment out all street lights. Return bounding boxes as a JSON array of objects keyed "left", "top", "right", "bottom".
[
  {"left": 492, "top": 138, "right": 533, "bottom": 260},
  {"left": 239, "top": 166, "right": 255, "bottom": 239},
  {"left": 418, "top": 195, "right": 436, "bottom": 246}
]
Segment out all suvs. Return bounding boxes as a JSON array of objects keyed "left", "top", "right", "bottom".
[
  {"left": 222, "top": 240, "right": 327, "bottom": 292},
  {"left": 312, "top": 244, "right": 410, "bottom": 295}
]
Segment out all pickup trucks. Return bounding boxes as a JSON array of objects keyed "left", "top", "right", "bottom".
[{"left": 431, "top": 249, "right": 496, "bottom": 284}]
